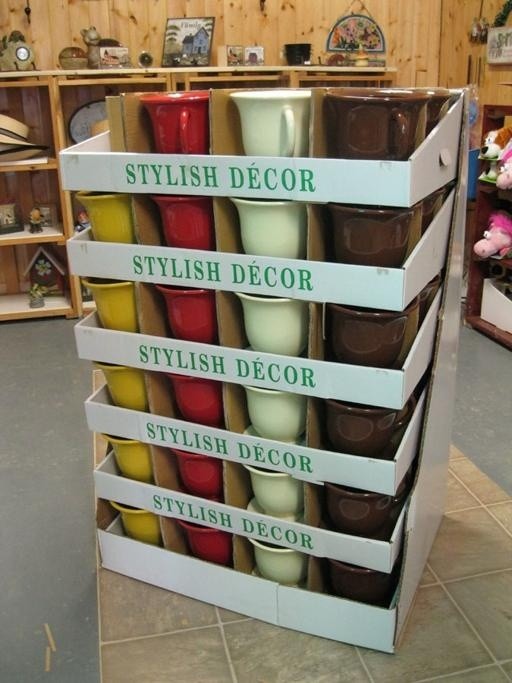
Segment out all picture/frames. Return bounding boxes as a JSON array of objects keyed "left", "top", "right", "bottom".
[{"left": 160, "top": 15, "right": 215, "bottom": 68}]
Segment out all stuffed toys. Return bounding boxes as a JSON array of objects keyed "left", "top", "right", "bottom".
[
  {"left": 473, "top": 211, "right": 512, "bottom": 259},
  {"left": 480, "top": 126, "right": 512, "bottom": 191}
]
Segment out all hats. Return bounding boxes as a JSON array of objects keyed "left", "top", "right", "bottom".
[{"left": 0, "top": 115, "right": 50, "bottom": 161}]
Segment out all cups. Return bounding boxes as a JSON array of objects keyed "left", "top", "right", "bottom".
[
  {"left": 152, "top": 194, "right": 217, "bottom": 570},
  {"left": 227, "top": 89, "right": 310, "bottom": 155},
  {"left": 228, "top": 193, "right": 307, "bottom": 593},
  {"left": 73, "top": 192, "right": 162, "bottom": 547},
  {"left": 320, "top": 192, "right": 423, "bottom": 611},
  {"left": 141, "top": 87, "right": 208, "bottom": 154},
  {"left": 284, "top": 43, "right": 311, "bottom": 64},
  {"left": 323, "top": 86, "right": 428, "bottom": 158}
]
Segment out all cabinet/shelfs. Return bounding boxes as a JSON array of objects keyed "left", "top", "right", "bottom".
[
  {"left": 0, "top": 71, "right": 395, "bottom": 324},
  {"left": 462, "top": 28, "right": 512, "bottom": 347}
]
